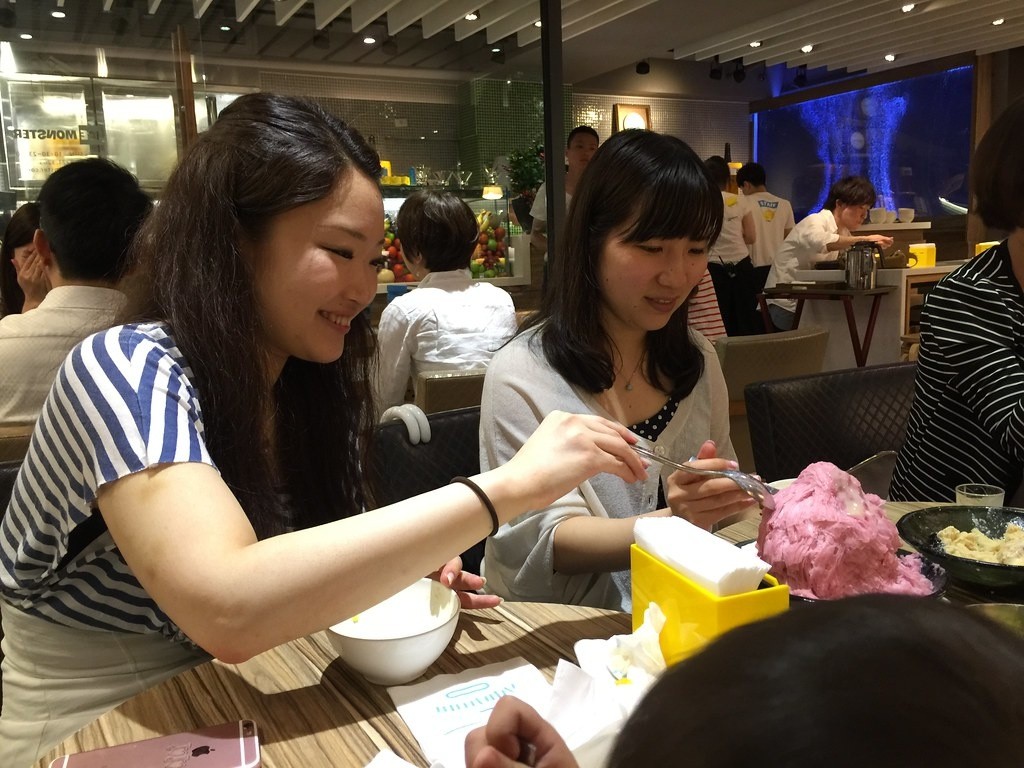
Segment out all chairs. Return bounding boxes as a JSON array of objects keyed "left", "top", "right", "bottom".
[{"left": 0, "top": 325, "right": 917, "bottom": 570}]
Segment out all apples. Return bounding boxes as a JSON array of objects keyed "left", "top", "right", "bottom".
[
  {"left": 382, "top": 238, "right": 418, "bottom": 282},
  {"left": 479, "top": 226, "right": 506, "bottom": 252}
]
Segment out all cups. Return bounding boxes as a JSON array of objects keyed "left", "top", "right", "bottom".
[
  {"left": 413, "top": 164, "right": 500, "bottom": 187},
  {"left": 956, "top": 483, "right": 1005, "bottom": 506}
]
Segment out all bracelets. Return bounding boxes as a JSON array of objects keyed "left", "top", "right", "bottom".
[{"left": 451, "top": 477, "right": 499, "bottom": 537}]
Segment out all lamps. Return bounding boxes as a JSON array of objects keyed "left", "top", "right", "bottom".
[{"left": 482, "top": 183, "right": 504, "bottom": 199}]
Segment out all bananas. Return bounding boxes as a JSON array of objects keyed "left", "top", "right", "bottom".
[{"left": 477, "top": 210, "right": 492, "bottom": 231}]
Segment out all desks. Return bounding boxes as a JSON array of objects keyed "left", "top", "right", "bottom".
[
  {"left": 3, "top": 601, "right": 642, "bottom": 768},
  {"left": 667, "top": 498, "right": 1024, "bottom": 639}
]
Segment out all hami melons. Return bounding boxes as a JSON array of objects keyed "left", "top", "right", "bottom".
[{"left": 377, "top": 268, "right": 395, "bottom": 283}]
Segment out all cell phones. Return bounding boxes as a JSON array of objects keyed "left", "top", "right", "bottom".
[{"left": 48, "top": 718, "right": 261, "bottom": 768}]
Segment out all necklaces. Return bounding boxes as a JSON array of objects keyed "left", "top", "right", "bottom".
[{"left": 616, "top": 352, "right": 643, "bottom": 390}]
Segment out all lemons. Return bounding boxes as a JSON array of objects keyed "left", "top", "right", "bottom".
[{"left": 470, "top": 261, "right": 507, "bottom": 279}]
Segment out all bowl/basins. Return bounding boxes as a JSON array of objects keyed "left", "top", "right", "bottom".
[
  {"left": 734, "top": 537, "right": 947, "bottom": 603},
  {"left": 896, "top": 506, "right": 1024, "bottom": 595},
  {"left": 814, "top": 260, "right": 843, "bottom": 268},
  {"left": 325, "top": 577, "right": 461, "bottom": 686},
  {"left": 884, "top": 249, "right": 907, "bottom": 268},
  {"left": 869, "top": 208, "right": 914, "bottom": 223}
]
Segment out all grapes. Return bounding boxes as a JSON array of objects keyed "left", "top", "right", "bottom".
[{"left": 482, "top": 244, "right": 503, "bottom": 269}]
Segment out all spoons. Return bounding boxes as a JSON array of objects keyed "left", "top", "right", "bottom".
[{"left": 629, "top": 442, "right": 776, "bottom": 511}]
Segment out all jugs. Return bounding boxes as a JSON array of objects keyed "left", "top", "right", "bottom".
[{"left": 843, "top": 241, "right": 886, "bottom": 289}]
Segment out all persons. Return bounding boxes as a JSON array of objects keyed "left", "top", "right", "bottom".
[
  {"left": 0, "top": 204, "right": 49, "bottom": 320},
  {"left": 735, "top": 163, "right": 795, "bottom": 292},
  {"left": 706, "top": 155, "right": 759, "bottom": 335},
  {"left": 757, "top": 176, "right": 894, "bottom": 330},
  {"left": 0, "top": 157, "right": 152, "bottom": 424},
  {"left": 0, "top": 90, "right": 648, "bottom": 768},
  {"left": 359, "top": 191, "right": 519, "bottom": 431},
  {"left": 530, "top": 126, "right": 599, "bottom": 252},
  {"left": 464, "top": 595, "right": 1024, "bottom": 768},
  {"left": 889, "top": 94, "right": 1024, "bottom": 504},
  {"left": 688, "top": 269, "right": 727, "bottom": 342},
  {"left": 480, "top": 128, "right": 760, "bottom": 610}
]
